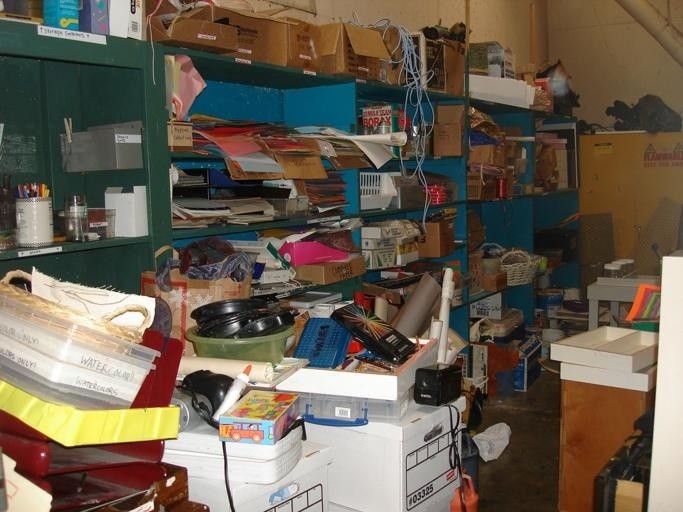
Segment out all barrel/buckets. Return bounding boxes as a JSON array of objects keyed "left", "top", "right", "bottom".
[{"left": 540, "top": 329, "right": 564, "bottom": 372}]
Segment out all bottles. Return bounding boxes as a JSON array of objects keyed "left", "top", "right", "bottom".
[{"left": 64, "top": 194, "right": 89, "bottom": 242}]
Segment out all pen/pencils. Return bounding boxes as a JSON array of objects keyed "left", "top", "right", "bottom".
[{"left": 15, "top": 181, "right": 50, "bottom": 198}]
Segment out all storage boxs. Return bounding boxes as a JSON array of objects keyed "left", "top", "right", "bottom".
[
  {"left": 496, "top": 339, "right": 543, "bottom": 393},
  {"left": 466, "top": 171, "right": 496, "bottom": 202},
  {"left": 482, "top": 271, "right": 508, "bottom": 293},
  {"left": 442, "top": 32, "right": 464, "bottom": 94},
  {"left": 469, "top": 144, "right": 505, "bottom": 167},
  {"left": 405, "top": 30, "right": 445, "bottom": 92},
  {"left": 147, "top": 2, "right": 236, "bottom": 50},
  {"left": 201, "top": 4, "right": 288, "bottom": 66},
  {"left": 317, "top": 21, "right": 371, "bottom": 76},
  {"left": 364, "top": 24, "right": 406, "bottom": 85},
  {"left": 278, "top": 16, "right": 323, "bottom": 69},
  {"left": 418, "top": 220, "right": 455, "bottom": 258},
  {"left": 179, "top": 391, "right": 470, "bottom": 512}
]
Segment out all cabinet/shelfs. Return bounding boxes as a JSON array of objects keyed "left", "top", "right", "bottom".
[{"left": 0, "top": 19, "right": 580, "bottom": 376}]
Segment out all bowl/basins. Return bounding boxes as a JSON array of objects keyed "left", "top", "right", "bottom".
[{"left": 184, "top": 298, "right": 296, "bottom": 367}]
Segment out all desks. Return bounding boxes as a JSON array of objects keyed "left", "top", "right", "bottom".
[{"left": 556, "top": 379, "right": 654, "bottom": 512}]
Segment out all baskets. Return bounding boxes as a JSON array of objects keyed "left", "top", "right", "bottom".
[
  {"left": 495, "top": 249, "right": 542, "bottom": 286},
  {"left": 421, "top": 185, "right": 447, "bottom": 206},
  {"left": 467, "top": 179, "right": 488, "bottom": 202},
  {"left": 0, "top": 269, "right": 150, "bottom": 355}
]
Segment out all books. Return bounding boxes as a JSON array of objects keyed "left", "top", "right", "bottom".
[
  {"left": 278, "top": 288, "right": 342, "bottom": 311},
  {"left": 166, "top": 110, "right": 409, "bottom": 234}
]
